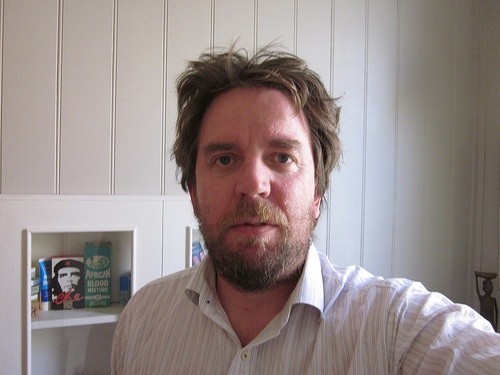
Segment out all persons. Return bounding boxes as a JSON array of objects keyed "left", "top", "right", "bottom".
[
  {"left": 475, "top": 276, "right": 499, "bottom": 334},
  {"left": 111, "top": 37, "right": 500, "bottom": 375}
]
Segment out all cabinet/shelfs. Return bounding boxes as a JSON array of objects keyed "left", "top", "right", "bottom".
[{"left": 0, "top": 194, "right": 211, "bottom": 375}]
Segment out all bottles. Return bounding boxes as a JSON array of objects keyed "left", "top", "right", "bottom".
[{"left": 119, "top": 275, "right": 128, "bottom": 305}]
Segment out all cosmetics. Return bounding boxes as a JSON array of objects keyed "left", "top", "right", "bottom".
[
  {"left": 119, "top": 275, "right": 129, "bottom": 306},
  {"left": 39, "top": 256, "right": 53, "bottom": 311}
]
